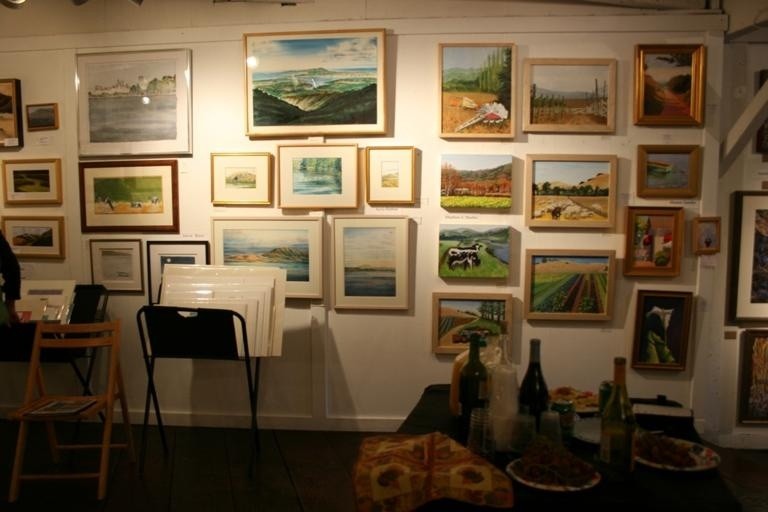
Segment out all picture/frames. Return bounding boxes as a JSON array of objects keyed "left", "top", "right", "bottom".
[
  {"left": 210, "top": 216, "right": 324, "bottom": 300},
  {"left": 74, "top": 46, "right": 192, "bottom": 159},
  {"left": 243, "top": 27, "right": 388, "bottom": 137},
  {"left": 331, "top": 215, "right": 411, "bottom": 311},
  {"left": 524, "top": 153, "right": 617, "bottom": 228},
  {"left": 275, "top": 144, "right": 359, "bottom": 211},
  {"left": 523, "top": 246, "right": 617, "bottom": 322},
  {"left": 431, "top": 292, "right": 513, "bottom": 354},
  {"left": 0, "top": 79, "right": 66, "bottom": 260},
  {"left": 365, "top": 145, "right": 415, "bottom": 205},
  {"left": 436, "top": 38, "right": 515, "bottom": 139},
  {"left": 210, "top": 153, "right": 273, "bottom": 206},
  {"left": 521, "top": 56, "right": 617, "bottom": 134},
  {"left": 89, "top": 239, "right": 144, "bottom": 295},
  {"left": 79, "top": 161, "right": 178, "bottom": 233},
  {"left": 147, "top": 240, "right": 211, "bottom": 305}
]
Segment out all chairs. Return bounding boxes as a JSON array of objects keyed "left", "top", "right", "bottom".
[
  {"left": 137, "top": 306, "right": 263, "bottom": 462},
  {"left": 1, "top": 283, "right": 110, "bottom": 425},
  {"left": 6, "top": 312, "right": 137, "bottom": 501}
]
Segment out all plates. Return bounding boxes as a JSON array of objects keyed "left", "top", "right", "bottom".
[
  {"left": 549, "top": 389, "right": 601, "bottom": 412},
  {"left": 634, "top": 437, "right": 721, "bottom": 471},
  {"left": 506, "top": 459, "right": 601, "bottom": 492}
]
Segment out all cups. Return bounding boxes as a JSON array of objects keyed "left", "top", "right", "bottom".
[
  {"left": 467, "top": 407, "right": 495, "bottom": 465},
  {"left": 540, "top": 410, "right": 561, "bottom": 438},
  {"left": 513, "top": 415, "right": 537, "bottom": 450}
]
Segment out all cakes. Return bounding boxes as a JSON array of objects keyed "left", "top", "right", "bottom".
[{"left": 549, "top": 385, "right": 598, "bottom": 409}]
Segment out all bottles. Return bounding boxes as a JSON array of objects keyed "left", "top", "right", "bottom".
[
  {"left": 600, "top": 357, "right": 635, "bottom": 481},
  {"left": 519, "top": 340, "right": 548, "bottom": 434},
  {"left": 458, "top": 333, "right": 487, "bottom": 431}
]
